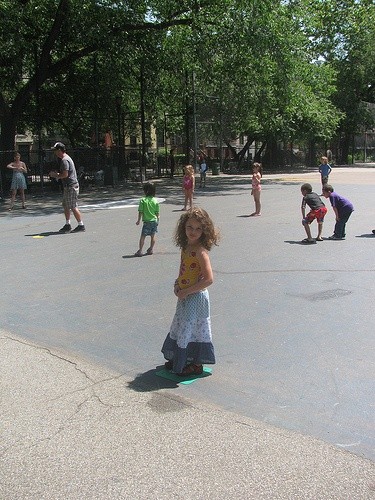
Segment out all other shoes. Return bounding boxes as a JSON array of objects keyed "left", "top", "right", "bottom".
[
  {"left": 135, "top": 250, "right": 143, "bottom": 256},
  {"left": 147, "top": 248, "right": 152, "bottom": 255},
  {"left": 252, "top": 212, "right": 261, "bottom": 216},
  {"left": 316, "top": 237, "right": 323, "bottom": 241},
  {"left": 302, "top": 238, "right": 316, "bottom": 244},
  {"left": 328, "top": 235, "right": 346, "bottom": 239},
  {"left": 71, "top": 225, "right": 86, "bottom": 233},
  {"left": 176, "top": 363, "right": 203, "bottom": 375},
  {"left": 59, "top": 224, "right": 72, "bottom": 232}
]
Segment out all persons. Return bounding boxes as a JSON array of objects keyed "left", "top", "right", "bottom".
[
  {"left": 161, "top": 208, "right": 224, "bottom": 375},
  {"left": 7, "top": 152, "right": 28, "bottom": 211},
  {"left": 182, "top": 165, "right": 194, "bottom": 211},
  {"left": 319, "top": 157, "right": 332, "bottom": 196},
  {"left": 301, "top": 183, "right": 327, "bottom": 242},
  {"left": 321, "top": 185, "right": 354, "bottom": 239},
  {"left": 46, "top": 143, "right": 85, "bottom": 234},
  {"left": 135, "top": 181, "right": 160, "bottom": 257},
  {"left": 250, "top": 162, "right": 262, "bottom": 216},
  {"left": 198, "top": 159, "right": 208, "bottom": 188}
]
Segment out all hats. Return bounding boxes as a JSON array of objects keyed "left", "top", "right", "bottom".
[{"left": 51, "top": 142, "right": 65, "bottom": 150}]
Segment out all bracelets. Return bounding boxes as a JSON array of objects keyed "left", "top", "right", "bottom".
[{"left": 174, "top": 283, "right": 179, "bottom": 287}]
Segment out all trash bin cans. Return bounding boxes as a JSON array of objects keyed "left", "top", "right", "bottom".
[{"left": 212, "top": 162, "right": 220, "bottom": 175}]
